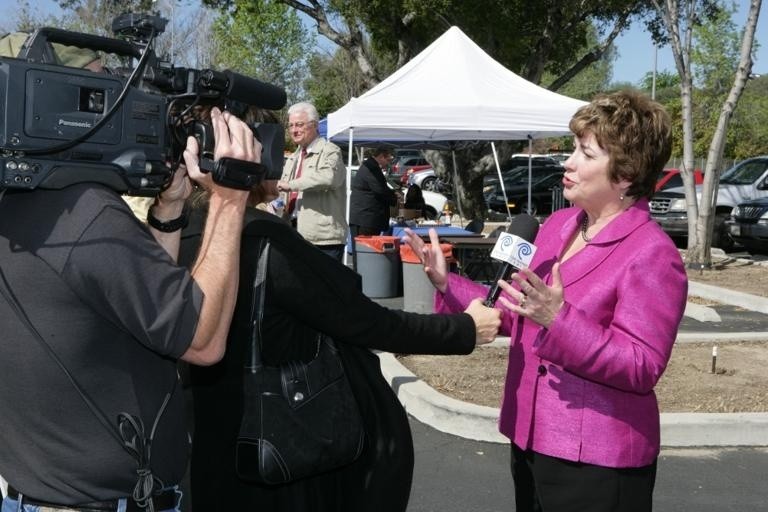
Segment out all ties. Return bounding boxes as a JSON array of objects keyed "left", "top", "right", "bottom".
[{"left": 288, "top": 149, "right": 306, "bottom": 213}]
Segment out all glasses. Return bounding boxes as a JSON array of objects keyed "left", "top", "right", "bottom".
[{"left": 288, "top": 121, "right": 314, "bottom": 126}]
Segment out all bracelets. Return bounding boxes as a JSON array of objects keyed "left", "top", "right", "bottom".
[{"left": 145, "top": 207, "right": 188, "bottom": 235}]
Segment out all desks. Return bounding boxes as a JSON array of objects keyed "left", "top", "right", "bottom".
[
  {"left": 439, "top": 236, "right": 498, "bottom": 283},
  {"left": 391, "top": 227, "right": 485, "bottom": 280}
]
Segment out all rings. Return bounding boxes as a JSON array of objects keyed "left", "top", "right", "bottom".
[{"left": 520, "top": 296, "right": 528, "bottom": 307}]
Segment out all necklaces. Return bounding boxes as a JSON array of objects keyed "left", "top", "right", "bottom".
[{"left": 582, "top": 218, "right": 593, "bottom": 243}]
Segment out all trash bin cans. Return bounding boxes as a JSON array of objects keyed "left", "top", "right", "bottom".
[
  {"left": 399, "top": 243, "right": 454, "bottom": 316},
  {"left": 354, "top": 235, "right": 401, "bottom": 299}
]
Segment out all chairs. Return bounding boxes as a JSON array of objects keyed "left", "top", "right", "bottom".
[{"left": 464, "top": 219, "right": 506, "bottom": 284}]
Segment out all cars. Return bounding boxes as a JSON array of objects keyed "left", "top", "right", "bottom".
[
  {"left": 344, "top": 165, "right": 449, "bottom": 219},
  {"left": 730, "top": 196, "right": 767, "bottom": 246}
]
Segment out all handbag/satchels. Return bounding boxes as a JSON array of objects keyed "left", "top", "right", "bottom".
[{"left": 233, "top": 334, "right": 366, "bottom": 485}]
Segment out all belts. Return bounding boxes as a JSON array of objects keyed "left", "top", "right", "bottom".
[{"left": 8, "top": 486, "right": 177, "bottom": 512}]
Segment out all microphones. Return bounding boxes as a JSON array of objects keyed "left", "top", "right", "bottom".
[{"left": 482, "top": 213, "right": 539, "bottom": 307}]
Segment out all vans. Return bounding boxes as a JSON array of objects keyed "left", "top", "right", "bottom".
[{"left": 648, "top": 155, "right": 768, "bottom": 250}]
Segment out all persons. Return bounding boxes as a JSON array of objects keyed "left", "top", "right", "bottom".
[
  {"left": 405, "top": 184, "right": 436, "bottom": 221},
  {"left": 1, "top": 29, "right": 264, "bottom": 511},
  {"left": 178, "top": 99, "right": 502, "bottom": 512},
  {"left": 349, "top": 145, "right": 402, "bottom": 271},
  {"left": 402, "top": 90, "right": 688, "bottom": 512},
  {"left": 277, "top": 101, "right": 347, "bottom": 265}
]
